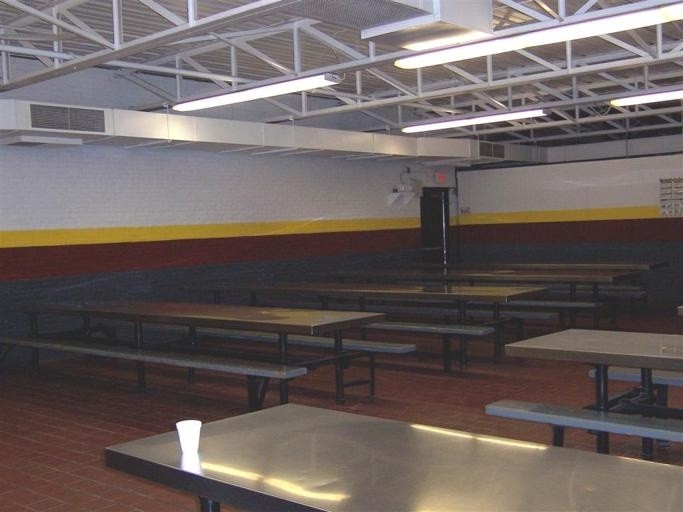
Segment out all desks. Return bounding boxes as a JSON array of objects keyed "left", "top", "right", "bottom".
[{"left": 104, "top": 401, "right": 683, "bottom": 512}]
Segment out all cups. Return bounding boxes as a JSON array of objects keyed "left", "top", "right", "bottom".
[
  {"left": 173, "top": 418, "right": 205, "bottom": 453},
  {"left": 180, "top": 453, "right": 203, "bottom": 479}
]
